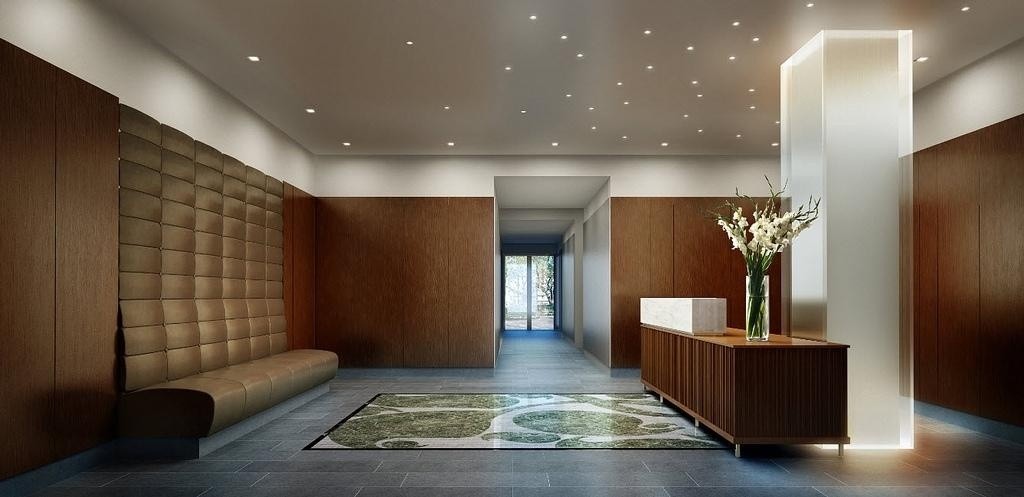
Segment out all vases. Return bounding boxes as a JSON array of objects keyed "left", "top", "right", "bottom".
[{"left": 744, "top": 272, "right": 770, "bottom": 341}]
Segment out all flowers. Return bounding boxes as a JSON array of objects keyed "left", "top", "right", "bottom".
[{"left": 702, "top": 174, "right": 822, "bottom": 342}]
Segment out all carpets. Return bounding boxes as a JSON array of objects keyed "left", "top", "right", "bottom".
[{"left": 303, "top": 390, "right": 726, "bottom": 453}]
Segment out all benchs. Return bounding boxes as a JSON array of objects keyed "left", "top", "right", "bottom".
[{"left": 102, "top": 343, "right": 340, "bottom": 461}]
